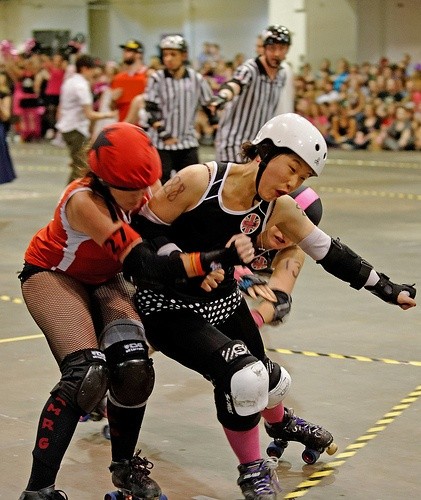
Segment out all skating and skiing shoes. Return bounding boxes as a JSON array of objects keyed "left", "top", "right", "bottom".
[
  {"left": 264, "top": 407, "right": 339, "bottom": 464},
  {"left": 105, "top": 449, "right": 169, "bottom": 500},
  {"left": 237, "top": 456, "right": 283, "bottom": 500},
  {"left": 17, "top": 485, "right": 68, "bottom": 500}
]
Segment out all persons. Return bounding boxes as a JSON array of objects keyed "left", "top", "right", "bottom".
[
  {"left": 79, "top": 185, "right": 323, "bottom": 440},
  {"left": 0, "top": 32, "right": 421, "bottom": 151},
  {"left": 15, "top": 123, "right": 256, "bottom": 500},
  {"left": 137, "top": 34, "right": 221, "bottom": 187},
  {"left": 0, "top": 71, "right": 17, "bottom": 185},
  {"left": 109, "top": 39, "right": 147, "bottom": 123},
  {"left": 204, "top": 25, "right": 291, "bottom": 164},
  {"left": 54, "top": 55, "right": 119, "bottom": 186},
  {"left": 130, "top": 112, "right": 416, "bottom": 500}
]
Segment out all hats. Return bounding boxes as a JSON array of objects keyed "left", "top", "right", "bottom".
[
  {"left": 120, "top": 40, "right": 144, "bottom": 54},
  {"left": 76, "top": 54, "right": 104, "bottom": 69}
]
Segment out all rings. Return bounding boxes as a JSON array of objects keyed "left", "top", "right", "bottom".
[{"left": 248, "top": 248, "right": 253, "bottom": 255}]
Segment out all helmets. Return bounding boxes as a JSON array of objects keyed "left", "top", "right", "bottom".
[
  {"left": 250, "top": 113, "right": 329, "bottom": 178},
  {"left": 262, "top": 24, "right": 292, "bottom": 49},
  {"left": 157, "top": 34, "right": 186, "bottom": 51},
  {"left": 288, "top": 184, "right": 323, "bottom": 225},
  {"left": 86, "top": 121, "right": 163, "bottom": 191}
]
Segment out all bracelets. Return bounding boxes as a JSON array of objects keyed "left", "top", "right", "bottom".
[{"left": 188, "top": 252, "right": 205, "bottom": 277}]
